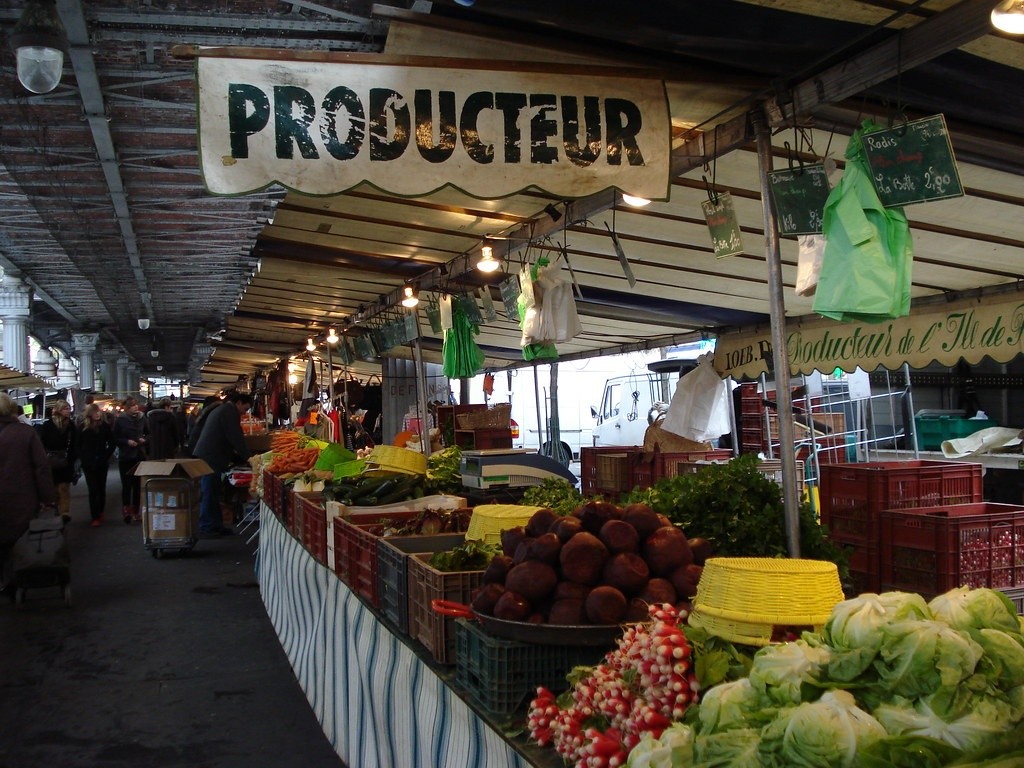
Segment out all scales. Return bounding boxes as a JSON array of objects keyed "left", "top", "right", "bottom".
[{"left": 460, "top": 448, "right": 579, "bottom": 507}]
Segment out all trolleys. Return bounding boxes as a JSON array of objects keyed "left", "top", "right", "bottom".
[{"left": 145, "top": 476, "right": 199, "bottom": 559}]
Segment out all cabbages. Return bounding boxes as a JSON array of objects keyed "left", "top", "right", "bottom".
[{"left": 623, "top": 584, "right": 1024, "bottom": 768}]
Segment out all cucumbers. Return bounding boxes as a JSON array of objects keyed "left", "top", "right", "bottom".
[{"left": 318, "top": 471, "right": 425, "bottom": 506}]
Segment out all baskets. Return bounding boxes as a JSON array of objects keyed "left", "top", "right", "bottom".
[
  {"left": 688, "top": 557, "right": 846, "bottom": 649},
  {"left": 457, "top": 402, "right": 512, "bottom": 431}
]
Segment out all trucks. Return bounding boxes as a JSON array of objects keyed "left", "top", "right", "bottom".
[{"left": 449, "top": 361, "right": 678, "bottom": 466}]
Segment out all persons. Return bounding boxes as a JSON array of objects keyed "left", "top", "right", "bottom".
[
  {"left": 0, "top": 393, "right": 56, "bottom": 594},
  {"left": 144, "top": 397, "right": 180, "bottom": 460},
  {"left": 16, "top": 399, "right": 80, "bottom": 525},
  {"left": 176, "top": 406, "right": 187, "bottom": 450},
  {"left": 187, "top": 391, "right": 252, "bottom": 536},
  {"left": 114, "top": 397, "right": 151, "bottom": 522},
  {"left": 75, "top": 395, "right": 116, "bottom": 528},
  {"left": 305, "top": 401, "right": 334, "bottom": 443}
]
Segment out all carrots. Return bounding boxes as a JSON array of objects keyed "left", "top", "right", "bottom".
[{"left": 267, "top": 430, "right": 321, "bottom": 473}]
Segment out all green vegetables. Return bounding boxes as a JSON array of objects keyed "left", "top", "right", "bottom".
[
  {"left": 523, "top": 449, "right": 854, "bottom": 583},
  {"left": 422, "top": 445, "right": 466, "bottom": 485},
  {"left": 372, "top": 508, "right": 503, "bottom": 573}
]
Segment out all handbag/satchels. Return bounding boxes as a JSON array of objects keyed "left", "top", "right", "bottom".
[{"left": 47, "top": 451, "right": 66, "bottom": 469}]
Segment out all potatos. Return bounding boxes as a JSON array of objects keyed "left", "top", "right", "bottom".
[{"left": 471, "top": 498, "right": 704, "bottom": 622}]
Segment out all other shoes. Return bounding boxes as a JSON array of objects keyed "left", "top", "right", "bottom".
[
  {"left": 123, "top": 506, "right": 134, "bottom": 522},
  {"left": 135, "top": 515, "right": 142, "bottom": 522},
  {"left": 197, "top": 527, "right": 235, "bottom": 540},
  {"left": 92, "top": 513, "right": 104, "bottom": 526}
]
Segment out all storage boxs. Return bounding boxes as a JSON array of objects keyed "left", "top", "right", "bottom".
[
  {"left": 126, "top": 459, "right": 214, "bottom": 508},
  {"left": 326, "top": 494, "right": 468, "bottom": 571},
  {"left": 454, "top": 617, "right": 608, "bottom": 722},
  {"left": 437, "top": 404, "right": 512, "bottom": 451},
  {"left": 407, "top": 551, "right": 486, "bottom": 671},
  {"left": 262, "top": 469, "right": 325, "bottom": 564},
  {"left": 140, "top": 503, "right": 199, "bottom": 544},
  {"left": 332, "top": 510, "right": 464, "bottom": 608},
  {"left": 910, "top": 417, "right": 995, "bottom": 451},
  {"left": 377, "top": 536, "right": 468, "bottom": 635},
  {"left": 579, "top": 445, "right": 1024, "bottom": 618},
  {"left": 733, "top": 382, "right": 859, "bottom": 463}
]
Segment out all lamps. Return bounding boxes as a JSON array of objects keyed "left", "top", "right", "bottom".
[
  {"left": 137, "top": 314, "right": 150, "bottom": 330},
  {"left": 157, "top": 365, "right": 163, "bottom": 372},
  {"left": 8, "top": 0, "right": 70, "bottom": 93},
  {"left": 151, "top": 349, "right": 159, "bottom": 357}
]
